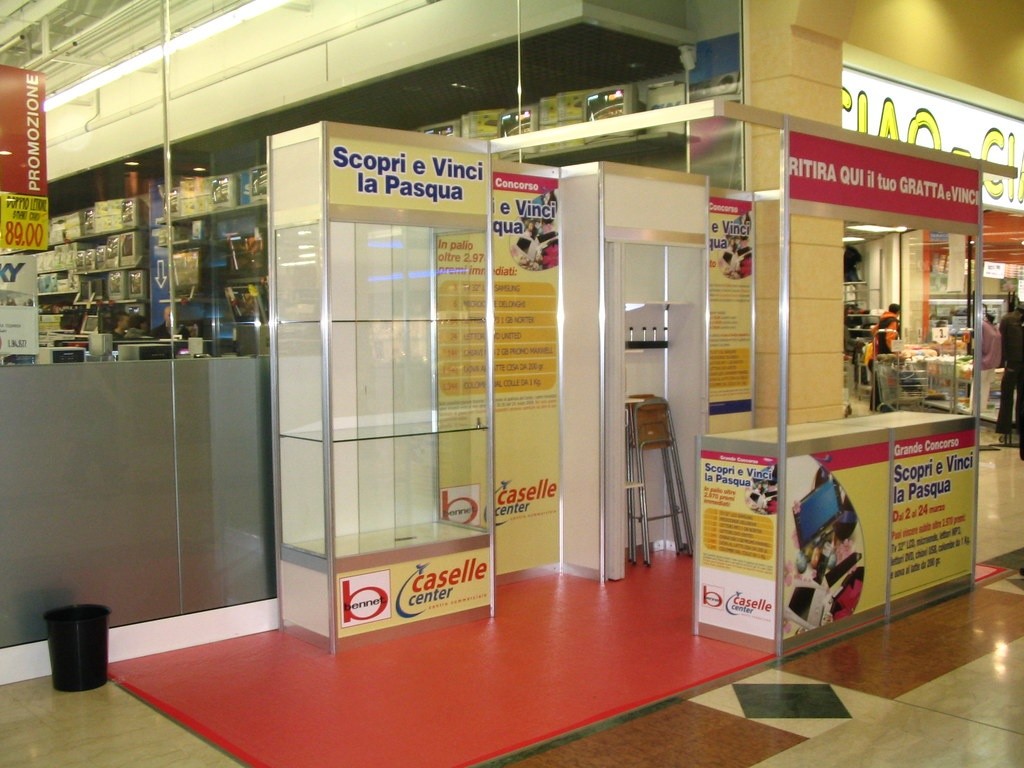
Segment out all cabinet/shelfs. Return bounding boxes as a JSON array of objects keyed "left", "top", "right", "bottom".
[
  {"left": 556, "top": 161, "right": 711, "bottom": 589},
  {"left": 845, "top": 282, "right": 1018, "bottom": 424},
  {"left": 264, "top": 120, "right": 497, "bottom": 657},
  {"left": 37, "top": 163, "right": 270, "bottom": 346}
]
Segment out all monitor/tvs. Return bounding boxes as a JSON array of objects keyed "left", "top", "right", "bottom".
[
  {"left": 158, "top": 164, "right": 267, "bottom": 324},
  {"left": 784, "top": 582, "right": 826, "bottom": 629},
  {"left": 76, "top": 197, "right": 151, "bottom": 335},
  {"left": 421, "top": 84, "right": 646, "bottom": 138}
]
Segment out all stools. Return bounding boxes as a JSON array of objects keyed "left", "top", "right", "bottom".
[{"left": 623, "top": 391, "right": 695, "bottom": 565}]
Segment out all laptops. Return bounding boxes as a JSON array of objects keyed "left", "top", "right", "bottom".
[{"left": 792, "top": 478, "right": 843, "bottom": 555}]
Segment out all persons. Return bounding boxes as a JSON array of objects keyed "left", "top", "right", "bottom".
[
  {"left": 970, "top": 299, "right": 1024, "bottom": 435},
  {"left": 863, "top": 303, "right": 900, "bottom": 410}
]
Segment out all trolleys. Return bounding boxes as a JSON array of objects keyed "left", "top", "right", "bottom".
[
  {"left": 876, "top": 354, "right": 933, "bottom": 414},
  {"left": 844, "top": 358, "right": 855, "bottom": 417}
]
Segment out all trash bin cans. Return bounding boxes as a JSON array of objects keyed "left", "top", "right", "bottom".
[{"left": 43, "top": 604, "right": 111, "bottom": 691}]
